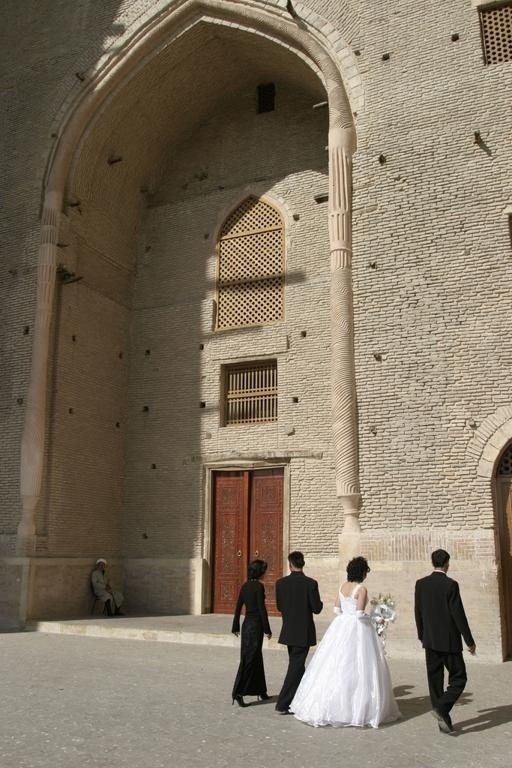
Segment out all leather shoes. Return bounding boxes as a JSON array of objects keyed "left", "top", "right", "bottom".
[{"left": 433, "top": 706, "right": 452, "bottom": 733}]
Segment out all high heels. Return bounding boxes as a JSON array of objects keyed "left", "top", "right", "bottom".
[
  {"left": 258, "top": 692, "right": 273, "bottom": 701},
  {"left": 232, "top": 691, "right": 251, "bottom": 707}
]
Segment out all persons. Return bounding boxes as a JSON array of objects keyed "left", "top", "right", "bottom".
[
  {"left": 287, "top": 555, "right": 404, "bottom": 728},
  {"left": 275, "top": 550, "right": 324, "bottom": 713},
  {"left": 414, "top": 548, "right": 477, "bottom": 734},
  {"left": 91, "top": 557, "right": 125, "bottom": 617},
  {"left": 231, "top": 560, "right": 273, "bottom": 708}
]
